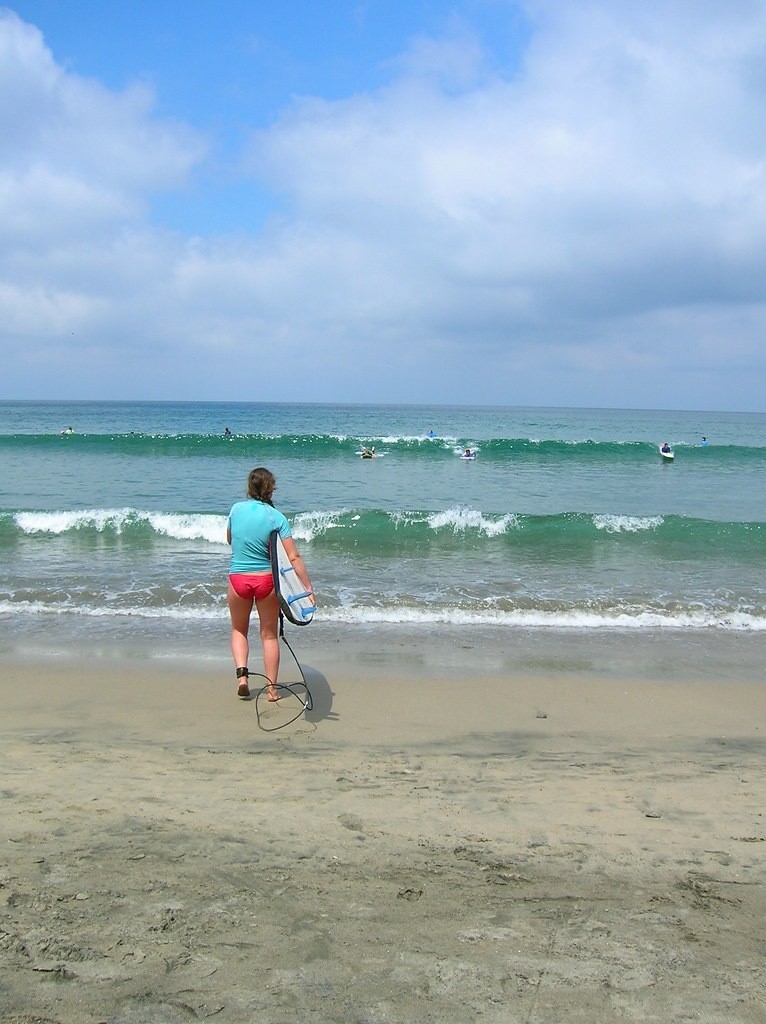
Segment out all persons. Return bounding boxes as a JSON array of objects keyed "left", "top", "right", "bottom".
[
  {"left": 701, "top": 438, "right": 708, "bottom": 446},
  {"left": 462, "top": 449, "right": 475, "bottom": 457},
  {"left": 662, "top": 443, "right": 670, "bottom": 453},
  {"left": 224, "top": 428, "right": 231, "bottom": 435},
  {"left": 65, "top": 427, "right": 74, "bottom": 435},
  {"left": 361, "top": 446, "right": 375, "bottom": 457},
  {"left": 429, "top": 431, "right": 435, "bottom": 437},
  {"left": 226, "top": 467, "right": 315, "bottom": 702}
]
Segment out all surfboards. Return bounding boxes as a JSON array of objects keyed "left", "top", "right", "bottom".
[{"left": 271, "top": 530, "right": 317, "bottom": 626}]
